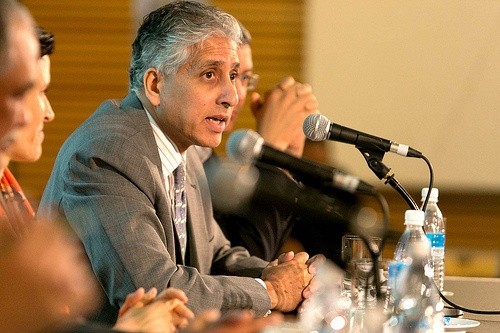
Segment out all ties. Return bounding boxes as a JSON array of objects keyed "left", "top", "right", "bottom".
[{"left": 173, "top": 162, "right": 188, "bottom": 266}]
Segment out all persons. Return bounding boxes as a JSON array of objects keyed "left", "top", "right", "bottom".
[
  {"left": 0, "top": 24, "right": 55, "bottom": 235},
  {"left": 0, "top": 0, "right": 196, "bottom": 333},
  {"left": 36, "top": 0, "right": 326, "bottom": 333},
  {"left": 193, "top": 20, "right": 321, "bottom": 279}
]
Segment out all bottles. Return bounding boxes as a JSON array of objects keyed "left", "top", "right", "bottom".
[
  {"left": 384, "top": 210, "right": 435, "bottom": 319},
  {"left": 420, "top": 187, "right": 446, "bottom": 292}
]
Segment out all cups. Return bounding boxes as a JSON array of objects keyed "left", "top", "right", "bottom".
[
  {"left": 350, "top": 259, "right": 377, "bottom": 309},
  {"left": 342, "top": 234, "right": 382, "bottom": 291}
]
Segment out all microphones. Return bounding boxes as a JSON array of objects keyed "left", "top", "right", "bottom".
[{"left": 209, "top": 114, "right": 423, "bottom": 233}]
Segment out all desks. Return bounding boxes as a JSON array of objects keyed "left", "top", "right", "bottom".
[{"left": 284, "top": 277, "right": 500, "bottom": 333}]
